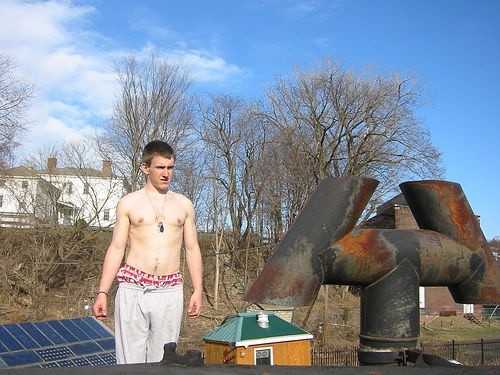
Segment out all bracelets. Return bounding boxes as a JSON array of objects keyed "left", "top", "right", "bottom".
[{"left": 97, "top": 291, "right": 108, "bottom": 296}]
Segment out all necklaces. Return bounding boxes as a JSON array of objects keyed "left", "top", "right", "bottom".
[{"left": 144, "top": 190, "right": 167, "bottom": 232}]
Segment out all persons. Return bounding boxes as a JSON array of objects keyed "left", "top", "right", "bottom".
[{"left": 92, "top": 141, "right": 203, "bottom": 364}]
